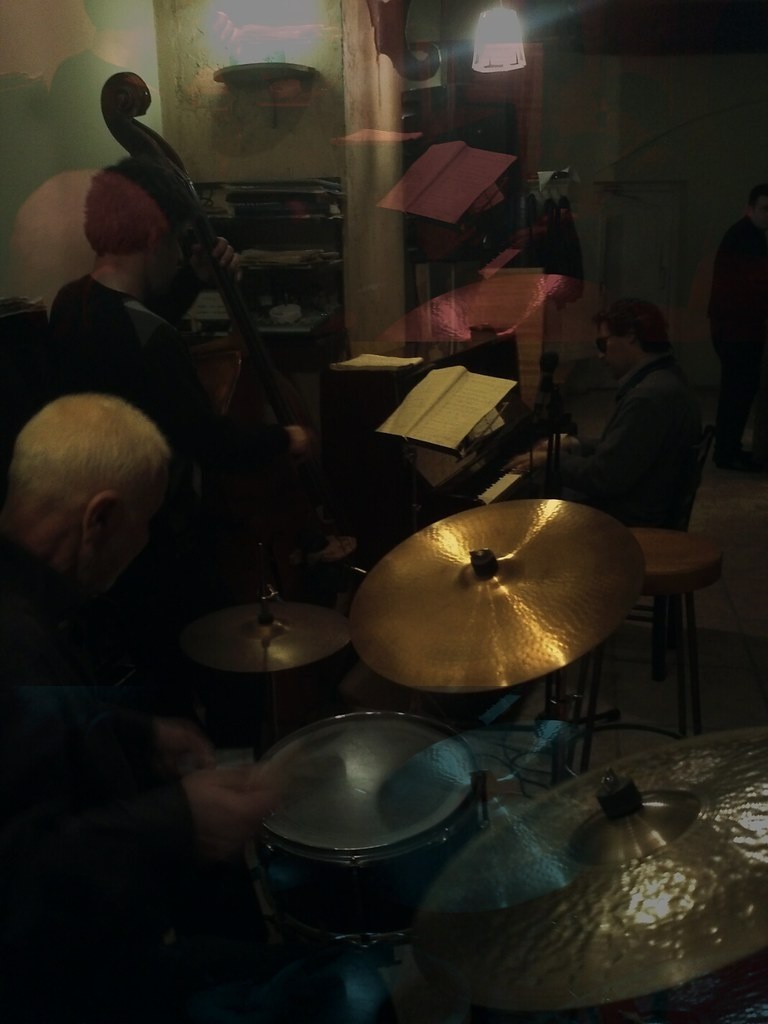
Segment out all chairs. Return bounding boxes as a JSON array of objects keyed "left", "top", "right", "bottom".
[{"left": 629, "top": 422, "right": 718, "bottom": 682}]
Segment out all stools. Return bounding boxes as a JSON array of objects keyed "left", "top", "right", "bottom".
[{"left": 567, "top": 527, "right": 723, "bottom": 775}]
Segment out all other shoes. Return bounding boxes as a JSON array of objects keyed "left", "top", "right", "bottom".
[{"left": 712, "top": 448, "right": 765, "bottom": 472}]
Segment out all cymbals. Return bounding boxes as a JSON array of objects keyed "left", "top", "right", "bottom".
[
  {"left": 349, "top": 498, "right": 647, "bottom": 692},
  {"left": 179, "top": 600, "right": 350, "bottom": 673},
  {"left": 407, "top": 725, "right": 767, "bottom": 1012}
]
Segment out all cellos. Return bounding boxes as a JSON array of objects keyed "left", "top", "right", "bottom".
[{"left": 99, "top": 74, "right": 359, "bottom": 600}]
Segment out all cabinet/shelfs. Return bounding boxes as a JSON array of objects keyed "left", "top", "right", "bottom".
[{"left": 186, "top": 178, "right": 345, "bottom": 343}]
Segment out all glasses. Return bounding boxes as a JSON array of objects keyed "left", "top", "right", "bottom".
[{"left": 596, "top": 333, "right": 615, "bottom": 354}]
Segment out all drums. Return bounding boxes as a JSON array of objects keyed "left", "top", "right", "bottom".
[{"left": 252, "top": 710, "right": 492, "bottom": 960}]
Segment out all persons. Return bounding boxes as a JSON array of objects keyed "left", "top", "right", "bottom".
[
  {"left": 503, "top": 303, "right": 704, "bottom": 528},
  {"left": 46, "top": 170, "right": 355, "bottom": 563},
  {"left": 708, "top": 176, "right": 768, "bottom": 473},
  {"left": 0, "top": 395, "right": 284, "bottom": 1015}
]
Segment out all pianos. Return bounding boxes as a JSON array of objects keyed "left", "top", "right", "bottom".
[{"left": 320, "top": 328, "right": 564, "bottom": 538}]
